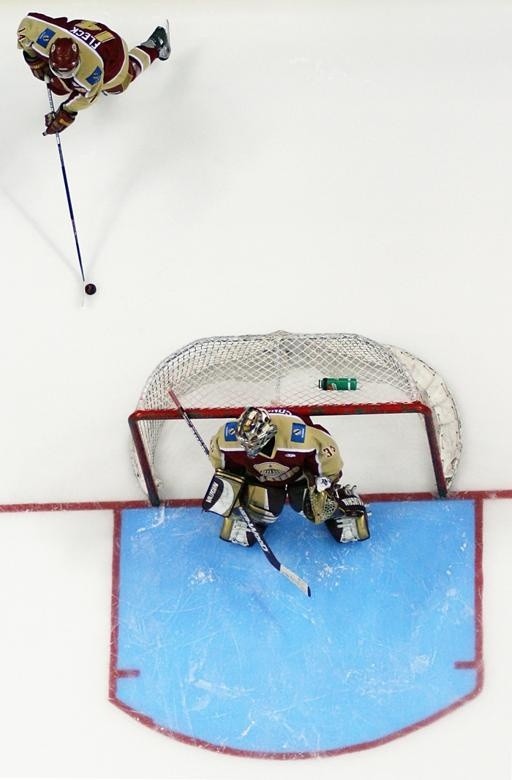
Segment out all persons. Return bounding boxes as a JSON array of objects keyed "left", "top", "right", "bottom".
[
  {"left": 15, "top": 12, "right": 170, "bottom": 136},
  {"left": 205, "top": 406, "right": 371, "bottom": 548}
]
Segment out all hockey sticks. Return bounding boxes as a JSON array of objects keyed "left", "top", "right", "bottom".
[
  {"left": 169, "top": 389, "right": 311, "bottom": 598},
  {"left": 43, "top": 74, "right": 86, "bottom": 307}
]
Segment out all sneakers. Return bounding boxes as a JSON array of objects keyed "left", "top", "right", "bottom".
[{"left": 153, "top": 26, "right": 171, "bottom": 61}]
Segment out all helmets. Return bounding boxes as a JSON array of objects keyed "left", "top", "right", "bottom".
[
  {"left": 233, "top": 406, "right": 276, "bottom": 459},
  {"left": 48, "top": 37, "right": 81, "bottom": 79}
]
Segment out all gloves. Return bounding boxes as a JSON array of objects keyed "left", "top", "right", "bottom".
[
  {"left": 42, "top": 102, "right": 78, "bottom": 136},
  {"left": 22, "top": 50, "right": 49, "bottom": 81}
]
